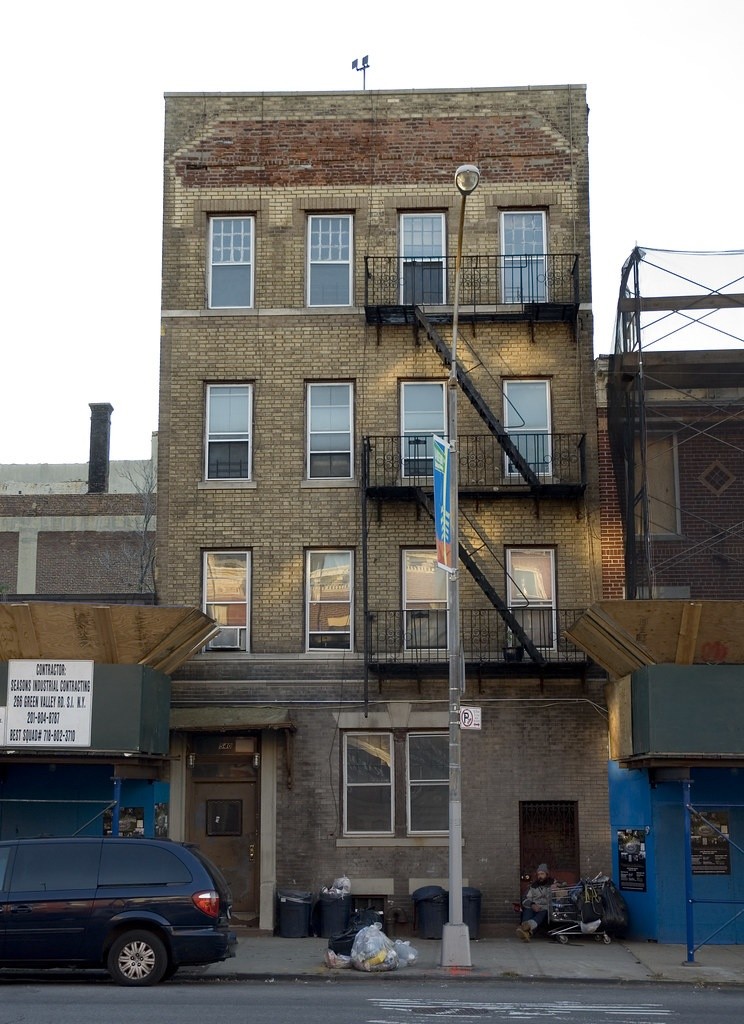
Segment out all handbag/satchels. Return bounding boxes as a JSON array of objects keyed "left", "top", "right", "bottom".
[{"left": 584, "top": 897, "right": 603, "bottom": 923}]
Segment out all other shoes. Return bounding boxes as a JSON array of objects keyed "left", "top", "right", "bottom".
[
  {"left": 516, "top": 928, "right": 531, "bottom": 943},
  {"left": 522, "top": 922, "right": 533, "bottom": 937}
]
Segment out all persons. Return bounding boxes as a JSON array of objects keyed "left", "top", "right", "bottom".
[{"left": 516, "top": 864, "right": 560, "bottom": 942}]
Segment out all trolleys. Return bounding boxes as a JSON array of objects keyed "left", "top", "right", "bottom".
[{"left": 545, "top": 876, "right": 612, "bottom": 944}]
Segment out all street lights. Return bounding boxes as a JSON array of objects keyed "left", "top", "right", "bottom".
[{"left": 432, "top": 166, "right": 483, "bottom": 970}]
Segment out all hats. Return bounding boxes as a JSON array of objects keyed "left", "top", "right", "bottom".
[{"left": 537, "top": 863, "right": 550, "bottom": 875}]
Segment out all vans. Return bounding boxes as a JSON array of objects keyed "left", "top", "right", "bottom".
[{"left": 1, "top": 835, "right": 238, "bottom": 987}]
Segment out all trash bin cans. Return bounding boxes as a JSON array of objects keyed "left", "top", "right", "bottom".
[
  {"left": 320, "top": 890, "right": 353, "bottom": 939},
  {"left": 462, "top": 886, "right": 483, "bottom": 940},
  {"left": 412, "top": 885, "right": 448, "bottom": 940},
  {"left": 276, "top": 888, "right": 313, "bottom": 938}
]
